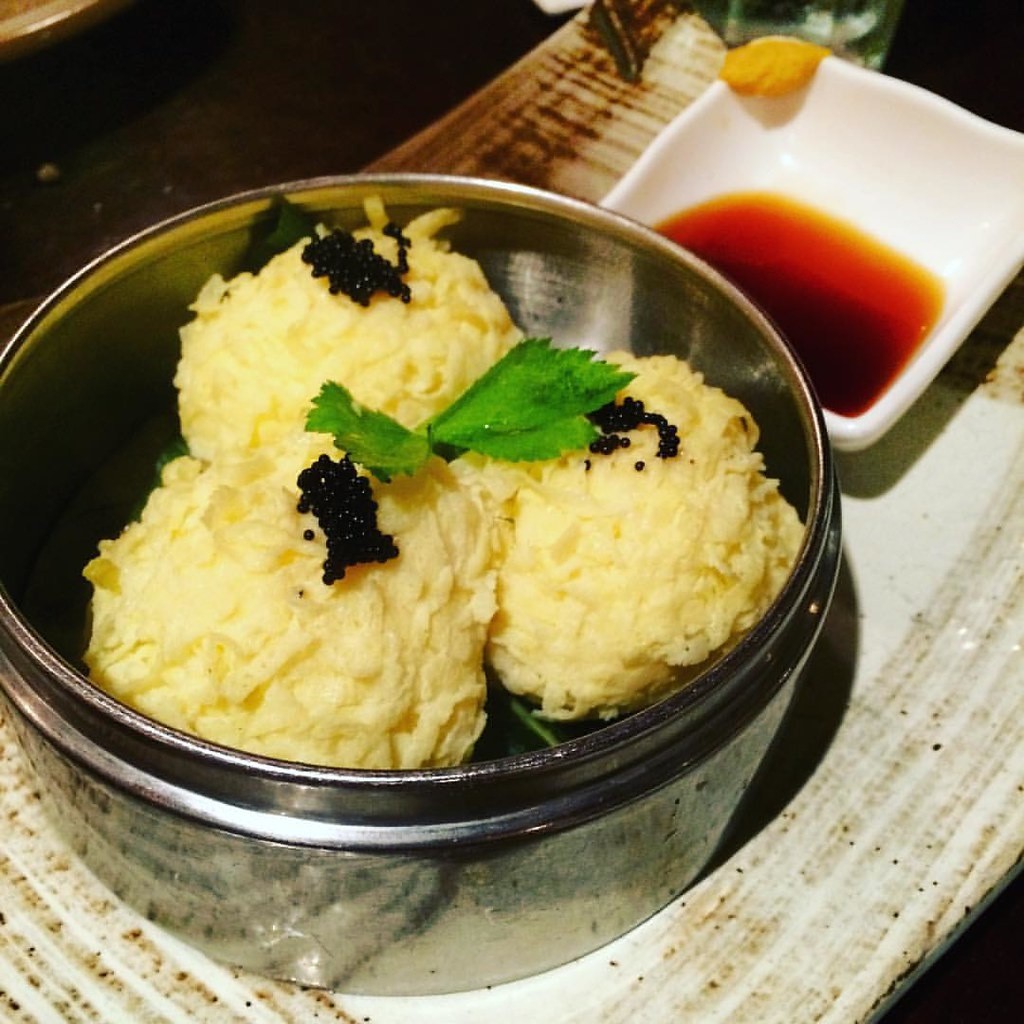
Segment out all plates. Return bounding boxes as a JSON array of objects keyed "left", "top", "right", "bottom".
[
  {"left": 0, "top": 0, "right": 1023, "bottom": 1024},
  {"left": 597, "top": 34, "right": 1024, "bottom": 449}
]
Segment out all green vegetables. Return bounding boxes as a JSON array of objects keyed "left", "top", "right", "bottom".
[{"left": 303, "top": 333, "right": 638, "bottom": 483}]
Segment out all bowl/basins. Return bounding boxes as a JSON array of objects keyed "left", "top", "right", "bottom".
[{"left": 1, "top": 178, "right": 844, "bottom": 997}]
